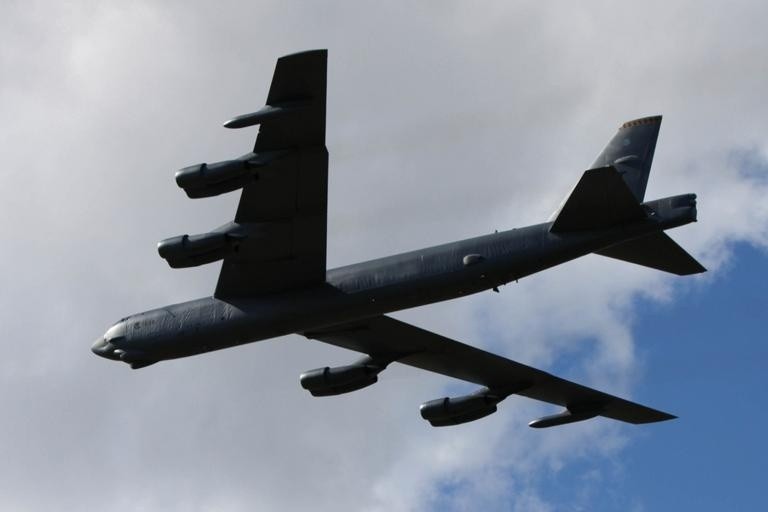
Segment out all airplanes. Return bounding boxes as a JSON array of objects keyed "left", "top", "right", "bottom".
[{"left": 88, "top": 46, "right": 709, "bottom": 426}]
